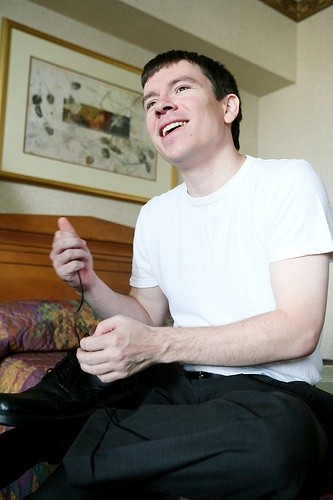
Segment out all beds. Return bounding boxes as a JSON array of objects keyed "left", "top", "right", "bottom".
[{"left": 0, "top": 213, "right": 173, "bottom": 500}]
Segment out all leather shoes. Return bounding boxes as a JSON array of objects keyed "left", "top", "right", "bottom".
[{"left": 0, "top": 348, "right": 155, "bottom": 427}]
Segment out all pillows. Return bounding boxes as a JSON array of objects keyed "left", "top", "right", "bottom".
[{"left": 0, "top": 300, "right": 174, "bottom": 356}]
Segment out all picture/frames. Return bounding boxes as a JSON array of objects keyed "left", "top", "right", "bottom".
[{"left": 0, "top": 16, "right": 179, "bottom": 205}]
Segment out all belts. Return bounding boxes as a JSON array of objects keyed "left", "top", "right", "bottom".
[{"left": 185, "top": 372, "right": 212, "bottom": 380}]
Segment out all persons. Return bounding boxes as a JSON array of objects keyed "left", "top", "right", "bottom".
[{"left": 0, "top": 49, "right": 333, "bottom": 500}]
